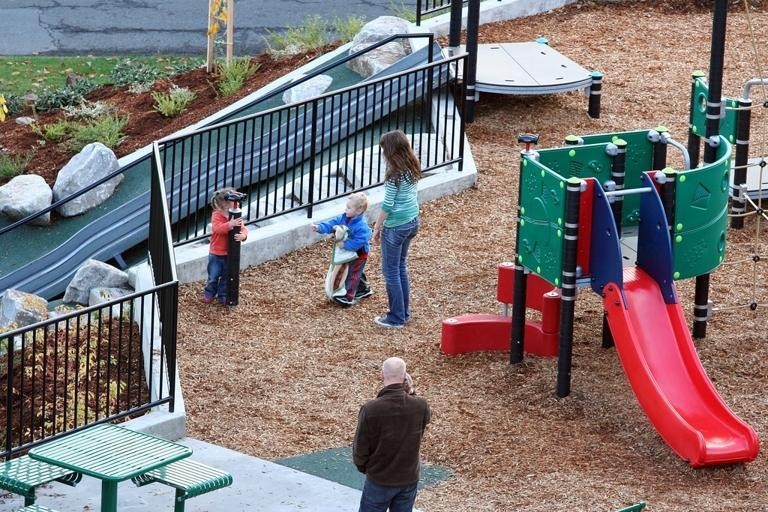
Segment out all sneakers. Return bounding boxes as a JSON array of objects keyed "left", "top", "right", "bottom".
[
  {"left": 333, "top": 296, "right": 355, "bottom": 306},
  {"left": 375, "top": 309, "right": 409, "bottom": 328},
  {"left": 203, "top": 294, "right": 228, "bottom": 305},
  {"left": 355, "top": 287, "right": 373, "bottom": 299}
]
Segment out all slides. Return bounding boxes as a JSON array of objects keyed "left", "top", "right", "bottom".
[{"left": 603, "top": 280, "right": 759, "bottom": 469}]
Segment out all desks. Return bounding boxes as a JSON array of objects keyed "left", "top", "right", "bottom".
[{"left": 29, "top": 424, "right": 193, "bottom": 512}]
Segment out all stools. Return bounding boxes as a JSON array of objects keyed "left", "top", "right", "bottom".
[
  {"left": 0, "top": 454, "right": 82, "bottom": 507},
  {"left": 16, "top": 503, "right": 58, "bottom": 512},
  {"left": 133, "top": 458, "right": 233, "bottom": 512}
]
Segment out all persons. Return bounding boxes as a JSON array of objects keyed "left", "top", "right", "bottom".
[
  {"left": 310, "top": 192, "right": 373, "bottom": 305},
  {"left": 198, "top": 187, "right": 249, "bottom": 305},
  {"left": 353, "top": 359, "right": 433, "bottom": 512},
  {"left": 370, "top": 131, "right": 423, "bottom": 328}
]
